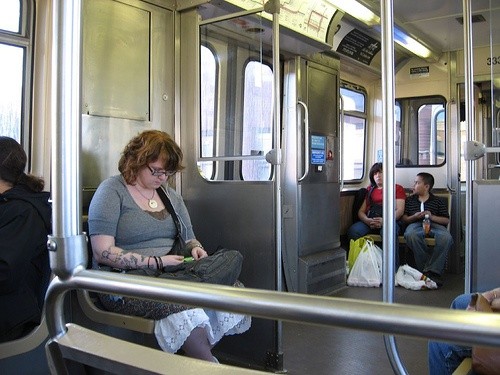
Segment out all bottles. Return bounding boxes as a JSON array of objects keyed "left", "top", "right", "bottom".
[{"left": 423, "top": 214, "right": 431, "bottom": 237}]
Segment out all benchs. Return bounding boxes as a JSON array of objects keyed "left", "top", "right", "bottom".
[{"left": 359, "top": 193, "right": 454, "bottom": 244}]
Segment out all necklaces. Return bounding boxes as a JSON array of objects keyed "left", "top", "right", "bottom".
[{"left": 131, "top": 182, "right": 158, "bottom": 208}]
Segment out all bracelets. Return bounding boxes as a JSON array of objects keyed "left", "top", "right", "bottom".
[{"left": 154, "top": 256, "right": 166, "bottom": 270}]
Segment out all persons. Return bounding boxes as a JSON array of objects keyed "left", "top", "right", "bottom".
[
  {"left": 346, "top": 164, "right": 407, "bottom": 274},
  {"left": 402, "top": 172, "right": 454, "bottom": 287},
  {"left": 86, "top": 130, "right": 251, "bottom": 364},
  {"left": 0, "top": 134, "right": 51, "bottom": 343},
  {"left": 429, "top": 288, "right": 500, "bottom": 375}
]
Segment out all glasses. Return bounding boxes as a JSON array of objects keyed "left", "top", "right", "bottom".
[{"left": 145, "top": 162, "right": 177, "bottom": 176}]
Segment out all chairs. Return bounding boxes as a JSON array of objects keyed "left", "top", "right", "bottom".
[
  {"left": 0, "top": 275, "right": 67, "bottom": 358},
  {"left": 77, "top": 213, "right": 173, "bottom": 335}
]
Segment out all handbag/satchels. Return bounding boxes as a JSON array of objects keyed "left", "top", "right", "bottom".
[
  {"left": 367, "top": 203, "right": 383, "bottom": 219},
  {"left": 395, "top": 263, "right": 437, "bottom": 291},
  {"left": 160, "top": 238, "right": 194, "bottom": 272},
  {"left": 346, "top": 236, "right": 384, "bottom": 287}
]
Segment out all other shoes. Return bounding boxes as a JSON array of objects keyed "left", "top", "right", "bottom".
[{"left": 423, "top": 270, "right": 443, "bottom": 285}]
